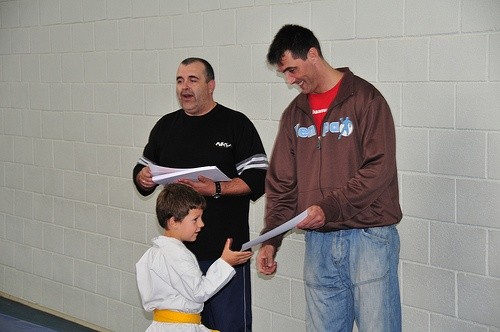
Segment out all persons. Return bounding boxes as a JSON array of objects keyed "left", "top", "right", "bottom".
[
  {"left": 133, "top": 57, "right": 270, "bottom": 332},
  {"left": 255, "top": 24, "right": 403, "bottom": 332},
  {"left": 135, "top": 183, "right": 255, "bottom": 332}
]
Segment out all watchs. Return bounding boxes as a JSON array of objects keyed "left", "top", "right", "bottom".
[{"left": 212, "top": 182, "right": 222, "bottom": 199}]
